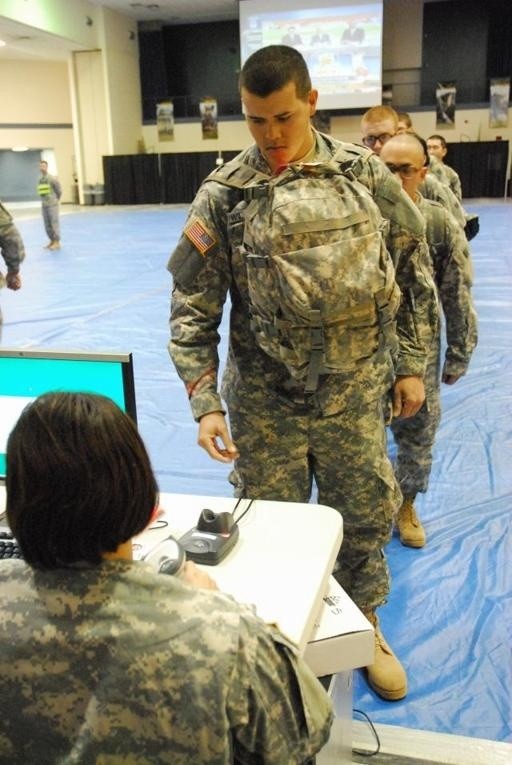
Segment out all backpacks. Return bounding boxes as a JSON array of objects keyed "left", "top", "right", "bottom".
[{"left": 201, "top": 142, "right": 404, "bottom": 394}]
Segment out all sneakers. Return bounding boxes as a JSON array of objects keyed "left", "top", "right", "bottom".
[
  {"left": 46, "top": 239, "right": 62, "bottom": 251},
  {"left": 393, "top": 501, "right": 426, "bottom": 549},
  {"left": 364, "top": 610, "right": 408, "bottom": 701}
]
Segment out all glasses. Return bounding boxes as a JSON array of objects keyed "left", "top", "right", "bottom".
[
  {"left": 385, "top": 161, "right": 421, "bottom": 180},
  {"left": 362, "top": 132, "right": 396, "bottom": 147}
]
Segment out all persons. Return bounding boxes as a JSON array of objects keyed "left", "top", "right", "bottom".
[
  {"left": 0, "top": 391, "right": 334, "bottom": 765},
  {"left": 37, "top": 161, "right": 63, "bottom": 251},
  {"left": 0, "top": 198, "right": 26, "bottom": 343},
  {"left": 169, "top": 47, "right": 439, "bottom": 702},
  {"left": 359, "top": 104, "right": 479, "bottom": 548}
]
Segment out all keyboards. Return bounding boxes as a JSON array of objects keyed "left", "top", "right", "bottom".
[{"left": 0, "top": 531, "right": 26, "bottom": 561}]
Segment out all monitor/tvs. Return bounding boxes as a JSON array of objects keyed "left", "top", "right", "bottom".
[{"left": 0, "top": 348, "right": 138, "bottom": 527}]
